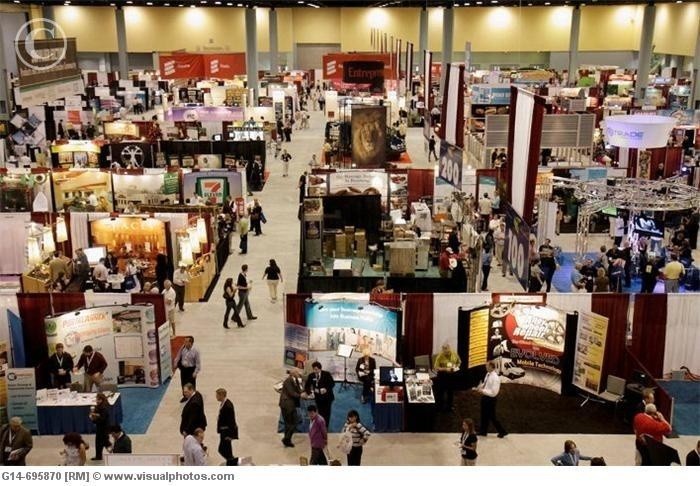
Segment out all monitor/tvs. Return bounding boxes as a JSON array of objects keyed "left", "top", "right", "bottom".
[
  {"left": 633, "top": 216, "right": 667, "bottom": 238},
  {"left": 323, "top": 192, "right": 383, "bottom": 230},
  {"left": 602, "top": 208, "right": 619, "bottom": 218},
  {"left": 378, "top": 365, "right": 403, "bottom": 385}
]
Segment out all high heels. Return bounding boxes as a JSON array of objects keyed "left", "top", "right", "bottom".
[{"left": 238, "top": 324, "right": 246, "bottom": 327}]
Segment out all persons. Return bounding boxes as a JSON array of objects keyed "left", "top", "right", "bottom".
[{"left": 0, "top": 78, "right": 700, "bottom": 466}]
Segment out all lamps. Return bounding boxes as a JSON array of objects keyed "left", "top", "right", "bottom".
[
  {"left": 27, "top": 236, "right": 43, "bottom": 279},
  {"left": 56, "top": 214, "right": 68, "bottom": 256},
  {"left": 43, "top": 222, "right": 56, "bottom": 262}
]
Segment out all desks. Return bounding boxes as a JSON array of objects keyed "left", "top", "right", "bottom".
[{"left": 37, "top": 392, "right": 122, "bottom": 435}]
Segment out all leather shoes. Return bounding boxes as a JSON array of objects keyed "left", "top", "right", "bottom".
[
  {"left": 282, "top": 439, "right": 294, "bottom": 447},
  {"left": 476, "top": 432, "right": 487, "bottom": 436},
  {"left": 498, "top": 432, "right": 508, "bottom": 438},
  {"left": 248, "top": 317, "right": 257, "bottom": 319},
  {"left": 181, "top": 397, "right": 188, "bottom": 402}
]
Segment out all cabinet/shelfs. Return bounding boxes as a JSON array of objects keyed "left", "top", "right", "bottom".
[{"left": 21, "top": 251, "right": 75, "bottom": 294}]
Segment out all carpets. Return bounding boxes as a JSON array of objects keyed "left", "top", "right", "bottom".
[
  {"left": 550, "top": 252, "right": 642, "bottom": 293},
  {"left": 117, "top": 376, "right": 172, "bottom": 434},
  {"left": 277, "top": 381, "right": 375, "bottom": 433}
]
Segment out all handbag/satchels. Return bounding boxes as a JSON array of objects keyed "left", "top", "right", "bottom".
[
  {"left": 221, "top": 426, "right": 239, "bottom": 439},
  {"left": 223, "top": 293, "right": 229, "bottom": 298},
  {"left": 336, "top": 432, "right": 353, "bottom": 454},
  {"left": 278, "top": 421, "right": 285, "bottom": 432}
]
